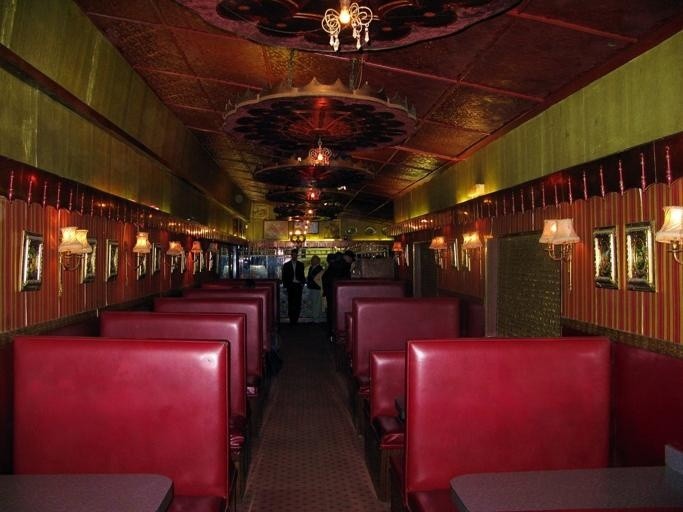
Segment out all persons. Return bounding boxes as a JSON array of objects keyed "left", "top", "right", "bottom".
[
  {"left": 322, "top": 250, "right": 358, "bottom": 338},
  {"left": 282, "top": 248, "right": 306, "bottom": 329},
  {"left": 302, "top": 256, "right": 323, "bottom": 324}
]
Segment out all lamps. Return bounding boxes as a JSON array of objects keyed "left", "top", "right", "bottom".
[
  {"left": 301, "top": 206, "right": 314, "bottom": 235},
  {"left": 462, "top": 230, "right": 485, "bottom": 279},
  {"left": 390, "top": 242, "right": 408, "bottom": 266},
  {"left": 540, "top": 217, "right": 579, "bottom": 271},
  {"left": 322, "top": 0, "right": 372, "bottom": 49},
  {"left": 304, "top": 184, "right": 321, "bottom": 200},
  {"left": 56, "top": 226, "right": 92, "bottom": 297},
  {"left": 654, "top": 206, "right": 682, "bottom": 266},
  {"left": 186, "top": 240, "right": 202, "bottom": 270},
  {"left": 163, "top": 241, "right": 184, "bottom": 280},
  {"left": 308, "top": 140, "right": 331, "bottom": 168},
  {"left": 429, "top": 236, "right": 446, "bottom": 269},
  {"left": 123, "top": 232, "right": 152, "bottom": 288}
]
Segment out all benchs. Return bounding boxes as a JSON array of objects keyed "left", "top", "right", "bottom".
[
  {"left": 332, "top": 279, "right": 611, "bottom": 512},
  {"left": 7, "top": 272, "right": 279, "bottom": 512}
]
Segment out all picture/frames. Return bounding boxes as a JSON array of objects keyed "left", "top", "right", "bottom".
[
  {"left": 135, "top": 243, "right": 146, "bottom": 280},
  {"left": 623, "top": 219, "right": 659, "bottom": 293},
  {"left": 448, "top": 238, "right": 460, "bottom": 271},
  {"left": 17, "top": 230, "right": 45, "bottom": 292},
  {"left": 103, "top": 239, "right": 119, "bottom": 283},
  {"left": 192, "top": 250, "right": 199, "bottom": 275},
  {"left": 79, "top": 238, "right": 97, "bottom": 284},
  {"left": 170, "top": 253, "right": 178, "bottom": 274},
  {"left": 151, "top": 242, "right": 160, "bottom": 275},
  {"left": 208, "top": 250, "right": 215, "bottom": 271},
  {"left": 591, "top": 225, "right": 621, "bottom": 290},
  {"left": 199, "top": 251, "right": 206, "bottom": 273},
  {"left": 180, "top": 254, "right": 186, "bottom": 274}
]
